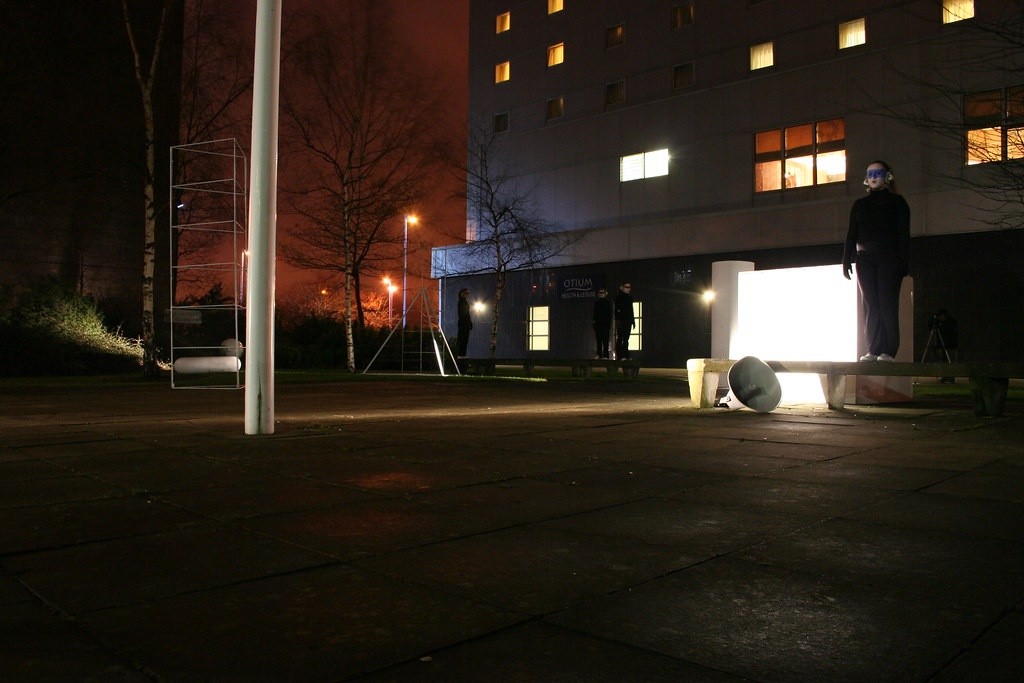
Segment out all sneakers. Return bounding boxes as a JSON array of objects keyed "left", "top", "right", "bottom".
[
  {"left": 859, "top": 354, "right": 878, "bottom": 361},
  {"left": 877, "top": 353, "right": 895, "bottom": 363}
]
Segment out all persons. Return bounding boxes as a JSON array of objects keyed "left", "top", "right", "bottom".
[
  {"left": 457, "top": 289, "right": 473, "bottom": 358},
  {"left": 842, "top": 160, "right": 911, "bottom": 362},
  {"left": 614, "top": 280, "right": 635, "bottom": 360},
  {"left": 592, "top": 288, "right": 613, "bottom": 360}
]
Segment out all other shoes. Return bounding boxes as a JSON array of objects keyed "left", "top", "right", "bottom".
[
  {"left": 594, "top": 355, "right": 602, "bottom": 360},
  {"left": 620, "top": 357, "right": 627, "bottom": 361},
  {"left": 457, "top": 355, "right": 470, "bottom": 359},
  {"left": 602, "top": 357, "right": 608, "bottom": 360},
  {"left": 627, "top": 358, "right": 633, "bottom": 361}
]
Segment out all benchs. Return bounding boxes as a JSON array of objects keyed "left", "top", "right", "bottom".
[
  {"left": 460, "top": 358, "right": 639, "bottom": 381},
  {"left": 686, "top": 357, "right": 1024, "bottom": 419}
]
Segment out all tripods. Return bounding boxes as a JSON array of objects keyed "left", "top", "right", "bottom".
[{"left": 918, "top": 321, "right": 952, "bottom": 365}]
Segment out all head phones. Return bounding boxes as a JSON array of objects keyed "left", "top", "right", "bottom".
[
  {"left": 604, "top": 288, "right": 609, "bottom": 295},
  {"left": 619, "top": 281, "right": 627, "bottom": 290},
  {"left": 460, "top": 289, "right": 464, "bottom": 297},
  {"left": 863, "top": 166, "right": 897, "bottom": 186}
]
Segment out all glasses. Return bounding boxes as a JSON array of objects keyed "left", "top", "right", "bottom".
[
  {"left": 624, "top": 286, "right": 631, "bottom": 288},
  {"left": 465, "top": 292, "right": 469, "bottom": 295},
  {"left": 600, "top": 291, "right": 606, "bottom": 294}
]
